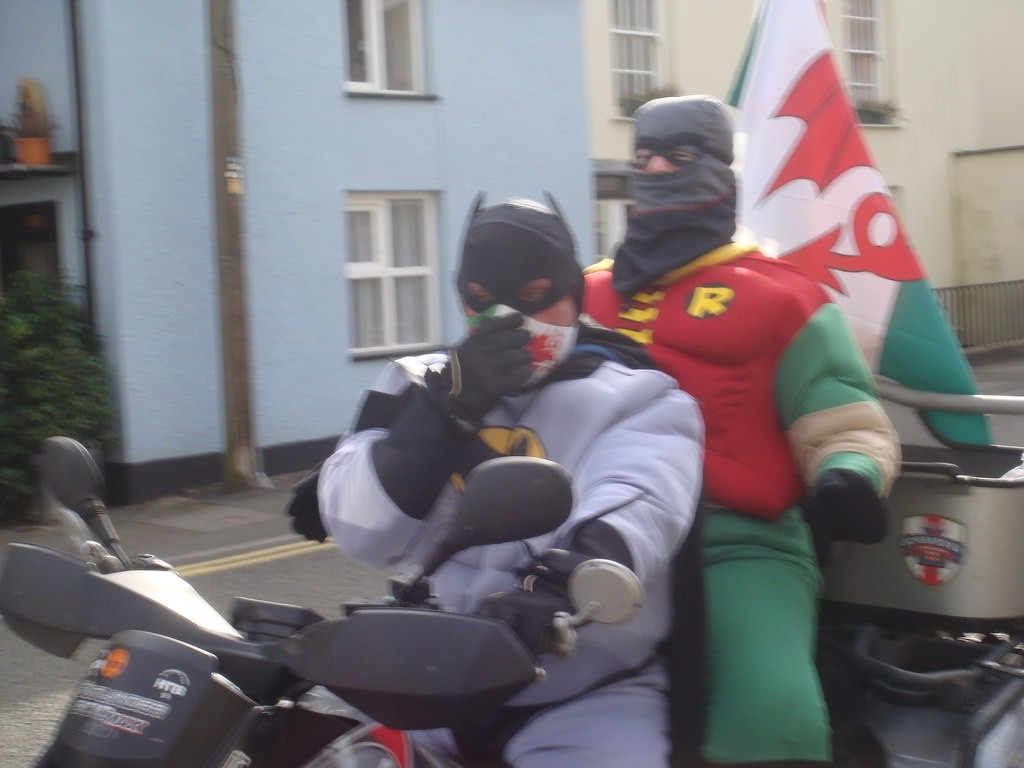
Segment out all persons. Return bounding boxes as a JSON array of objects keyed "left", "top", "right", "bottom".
[
  {"left": 317, "top": 191, "right": 705, "bottom": 768},
  {"left": 284, "top": 95, "right": 898, "bottom": 765}
]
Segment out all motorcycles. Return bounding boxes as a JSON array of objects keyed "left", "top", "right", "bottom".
[{"left": 0, "top": 332, "right": 1024, "bottom": 767}]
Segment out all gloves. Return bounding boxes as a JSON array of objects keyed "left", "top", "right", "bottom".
[
  {"left": 371, "top": 313, "right": 534, "bottom": 519},
  {"left": 286, "top": 460, "right": 328, "bottom": 544},
  {"left": 802, "top": 468, "right": 891, "bottom": 544},
  {"left": 476, "top": 518, "right": 634, "bottom": 653}
]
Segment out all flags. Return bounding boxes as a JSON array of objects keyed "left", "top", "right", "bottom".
[{"left": 723, "top": 1, "right": 993, "bottom": 446}]
